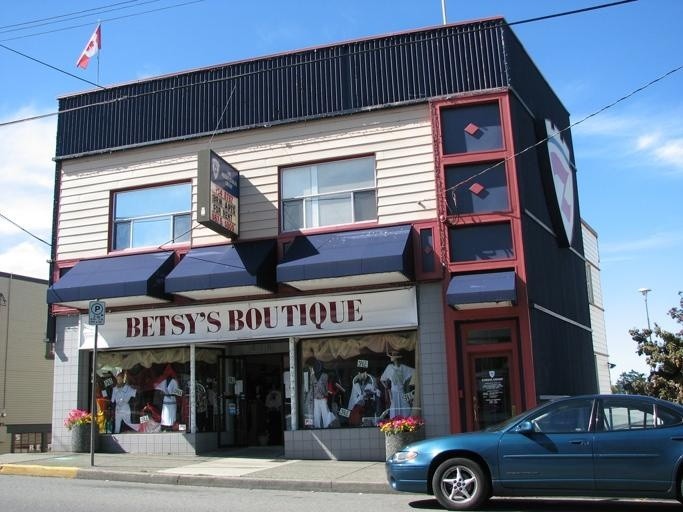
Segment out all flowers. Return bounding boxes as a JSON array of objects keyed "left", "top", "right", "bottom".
[
  {"left": 377, "top": 414, "right": 426, "bottom": 437},
  {"left": 63, "top": 409, "right": 92, "bottom": 432}
]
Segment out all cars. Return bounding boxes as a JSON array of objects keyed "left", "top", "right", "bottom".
[{"left": 385, "top": 395, "right": 682, "bottom": 510}]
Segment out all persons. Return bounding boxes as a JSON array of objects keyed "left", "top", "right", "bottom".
[
  {"left": 380, "top": 351, "right": 415, "bottom": 418},
  {"left": 309, "top": 363, "right": 336, "bottom": 428},
  {"left": 347, "top": 371, "right": 379, "bottom": 425},
  {"left": 154, "top": 376, "right": 177, "bottom": 427},
  {"left": 111, "top": 372, "right": 140, "bottom": 433}
]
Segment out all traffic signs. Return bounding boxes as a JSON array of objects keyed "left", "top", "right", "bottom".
[{"left": 88, "top": 301, "right": 104, "bottom": 325}]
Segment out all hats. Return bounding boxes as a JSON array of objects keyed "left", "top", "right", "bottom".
[{"left": 386, "top": 350, "right": 403, "bottom": 358}]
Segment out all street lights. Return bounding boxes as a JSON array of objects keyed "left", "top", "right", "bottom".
[{"left": 638, "top": 288, "right": 651, "bottom": 344}]
